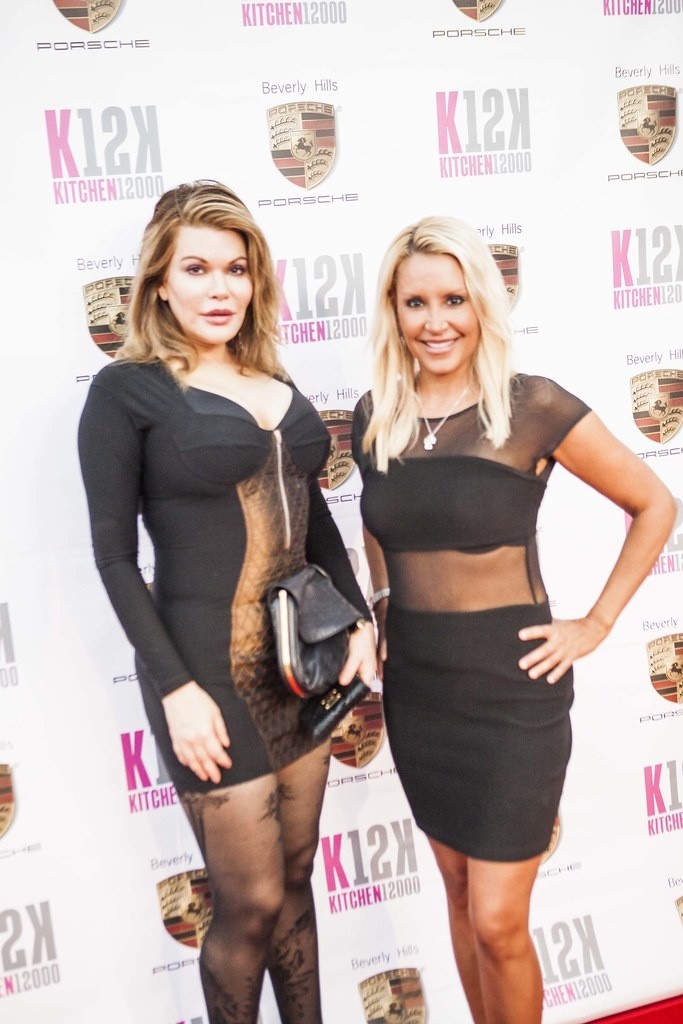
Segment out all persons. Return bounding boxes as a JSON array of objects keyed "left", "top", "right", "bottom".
[
  {"left": 77, "top": 183, "right": 378, "bottom": 1024},
  {"left": 351, "top": 215, "right": 676, "bottom": 1024}
]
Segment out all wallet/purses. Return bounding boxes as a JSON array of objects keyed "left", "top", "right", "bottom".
[{"left": 297, "top": 674, "right": 370, "bottom": 744}]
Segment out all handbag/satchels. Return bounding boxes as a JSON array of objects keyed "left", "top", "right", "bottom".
[{"left": 265, "top": 561, "right": 363, "bottom": 699}]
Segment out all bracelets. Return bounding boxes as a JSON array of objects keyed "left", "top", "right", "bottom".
[{"left": 370, "top": 587, "right": 390, "bottom": 609}]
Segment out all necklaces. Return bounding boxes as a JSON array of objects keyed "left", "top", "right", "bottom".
[{"left": 414, "top": 384, "right": 467, "bottom": 450}]
